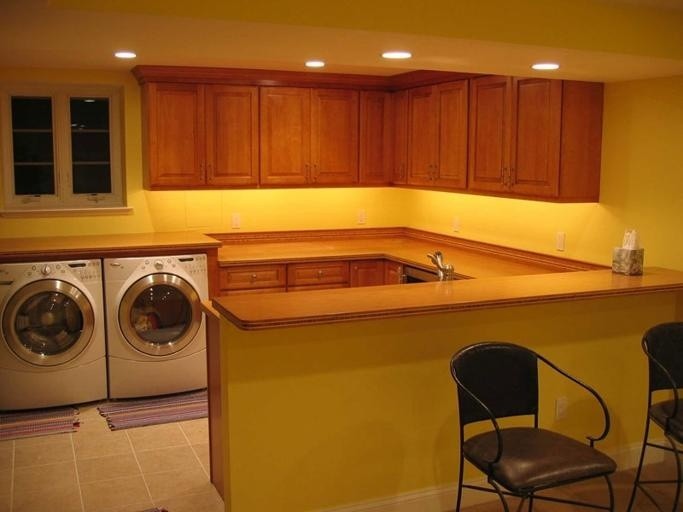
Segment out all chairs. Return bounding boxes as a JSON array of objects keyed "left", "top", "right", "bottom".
[
  {"left": 448, "top": 341, "right": 619, "bottom": 512},
  {"left": 626, "top": 321, "right": 683, "bottom": 512}
]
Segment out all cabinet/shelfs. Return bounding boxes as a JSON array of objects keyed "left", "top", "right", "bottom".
[
  {"left": 287, "top": 260, "right": 350, "bottom": 298},
  {"left": 406, "top": 71, "right": 469, "bottom": 191},
  {"left": 359, "top": 76, "right": 406, "bottom": 186},
  {"left": 259, "top": 72, "right": 362, "bottom": 190},
  {"left": 468, "top": 74, "right": 602, "bottom": 202},
  {"left": 140, "top": 62, "right": 260, "bottom": 190},
  {"left": 349, "top": 259, "right": 402, "bottom": 287},
  {"left": 219, "top": 262, "right": 287, "bottom": 304}
]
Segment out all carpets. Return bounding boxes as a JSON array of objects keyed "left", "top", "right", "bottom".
[
  {"left": 101, "top": 392, "right": 214, "bottom": 433},
  {"left": 0, "top": 403, "right": 79, "bottom": 443}
]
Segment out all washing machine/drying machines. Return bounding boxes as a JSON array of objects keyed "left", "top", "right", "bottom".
[
  {"left": 102, "top": 254, "right": 210, "bottom": 400},
  {"left": 0, "top": 259, "right": 108, "bottom": 410}
]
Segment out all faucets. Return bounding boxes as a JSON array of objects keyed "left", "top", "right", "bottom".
[{"left": 426, "top": 250, "right": 454, "bottom": 282}]
